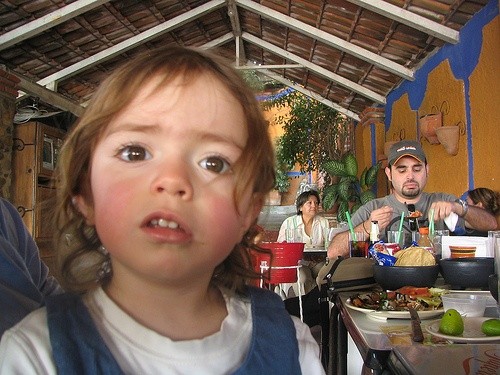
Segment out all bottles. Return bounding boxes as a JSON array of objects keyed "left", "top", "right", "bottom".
[
  {"left": 369, "top": 218, "right": 380, "bottom": 251},
  {"left": 414, "top": 228, "right": 436, "bottom": 262}
]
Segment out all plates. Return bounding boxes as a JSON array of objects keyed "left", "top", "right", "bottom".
[
  {"left": 345, "top": 295, "right": 446, "bottom": 318},
  {"left": 426, "top": 317, "right": 500, "bottom": 343}
]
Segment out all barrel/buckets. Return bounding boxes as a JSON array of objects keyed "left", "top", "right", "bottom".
[{"left": 258, "top": 240, "right": 305, "bottom": 283}]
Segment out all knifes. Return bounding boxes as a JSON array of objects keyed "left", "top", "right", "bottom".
[{"left": 407, "top": 303, "right": 424, "bottom": 343}]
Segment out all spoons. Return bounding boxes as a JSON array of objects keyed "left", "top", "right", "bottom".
[{"left": 387, "top": 212, "right": 421, "bottom": 218}]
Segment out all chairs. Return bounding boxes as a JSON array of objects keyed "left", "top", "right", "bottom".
[{"left": 258, "top": 241, "right": 305, "bottom": 322}]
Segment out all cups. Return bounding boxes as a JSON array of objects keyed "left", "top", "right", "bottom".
[
  {"left": 488, "top": 230, "right": 500, "bottom": 306},
  {"left": 449, "top": 245, "right": 476, "bottom": 259},
  {"left": 348, "top": 232, "right": 370, "bottom": 258},
  {"left": 386, "top": 231, "right": 405, "bottom": 251},
  {"left": 430, "top": 230, "right": 449, "bottom": 256},
  {"left": 412, "top": 231, "right": 419, "bottom": 247}
]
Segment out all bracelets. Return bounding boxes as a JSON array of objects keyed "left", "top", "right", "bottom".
[{"left": 362, "top": 222, "right": 370, "bottom": 236}]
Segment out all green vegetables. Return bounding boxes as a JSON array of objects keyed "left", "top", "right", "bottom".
[{"left": 379, "top": 292, "right": 394, "bottom": 310}]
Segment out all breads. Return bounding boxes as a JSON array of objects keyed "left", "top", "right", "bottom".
[
  {"left": 481, "top": 318, "right": 500, "bottom": 336},
  {"left": 393, "top": 246, "right": 436, "bottom": 267},
  {"left": 439, "top": 308, "right": 464, "bottom": 336}
]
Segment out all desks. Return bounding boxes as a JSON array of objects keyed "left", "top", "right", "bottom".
[
  {"left": 302, "top": 249, "right": 365, "bottom": 261},
  {"left": 328, "top": 275, "right": 500, "bottom": 375}
]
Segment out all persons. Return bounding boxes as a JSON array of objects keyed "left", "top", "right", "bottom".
[
  {"left": 275, "top": 189, "right": 332, "bottom": 300},
  {"left": 327, "top": 141, "right": 497, "bottom": 260},
  {"left": 0, "top": 44, "right": 327, "bottom": 375},
  {"left": 0, "top": 198, "right": 65, "bottom": 342},
  {"left": 466, "top": 188, "right": 500, "bottom": 214}
]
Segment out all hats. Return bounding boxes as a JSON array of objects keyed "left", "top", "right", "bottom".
[{"left": 388, "top": 141, "right": 425, "bottom": 166}]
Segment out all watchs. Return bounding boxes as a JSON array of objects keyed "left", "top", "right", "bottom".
[{"left": 456, "top": 198, "right": 468, "bottom": 218}]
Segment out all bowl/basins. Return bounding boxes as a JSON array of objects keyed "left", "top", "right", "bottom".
[
  {"left": 440, "top": 259, "right": 493, "bottom": 289},
  {"left": 373, "top": 263, "right": 438, "bottom": 291},
  {"left": 302, "top": 249, "right": 328, "bottom": 263}
]
